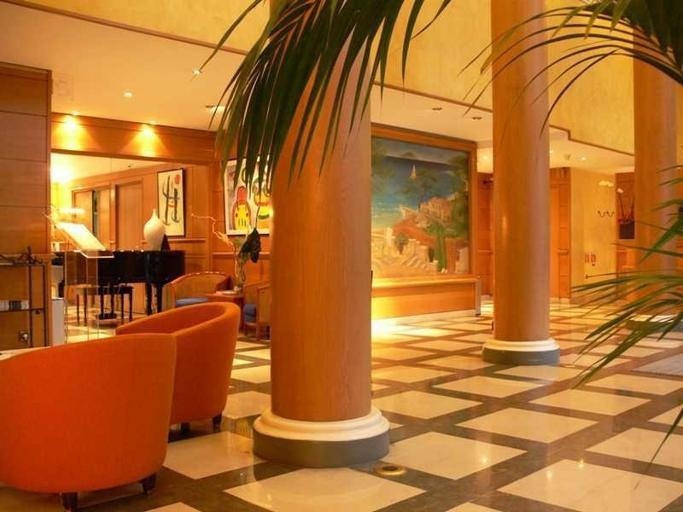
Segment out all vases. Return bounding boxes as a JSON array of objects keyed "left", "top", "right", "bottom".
[{"left": 143, "top": 208, "right": 165, "bottom": 252}]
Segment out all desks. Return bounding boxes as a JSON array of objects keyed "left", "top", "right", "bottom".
[
  {"left": 204, "top": 290, "right": 245, "bottom": 332},
  {"left": 58, "top": 250, "right": 186, "bottom": 316}
]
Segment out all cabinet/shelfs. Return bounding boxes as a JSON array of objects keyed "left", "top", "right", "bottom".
[{"left": 0, "top": 246, "right": 51, "bottom": 348}]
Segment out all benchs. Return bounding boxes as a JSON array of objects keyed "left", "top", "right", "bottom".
[{"left": 74, "top": 253, "right": 133, "bottom": 324}]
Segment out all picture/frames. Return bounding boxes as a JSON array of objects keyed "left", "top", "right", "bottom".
[
  {"left": 219, "top": 151, "right": 271, "bottom": 239},
  {"left": 154, "top": 167, "right": 187, "bottom": 241}
]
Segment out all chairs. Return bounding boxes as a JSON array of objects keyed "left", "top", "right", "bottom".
[
  {"left": 0, "top": 329, "right": 178, "bottom": 511},
  {"left": 240, "top": 277, "right": 272, "bottom": 344},
  {"left": 113, "top": 299, "right": 243, "bottom": 441},
  {"left": 161, "top": 270, "right": 233, "bottom": 314}
]
